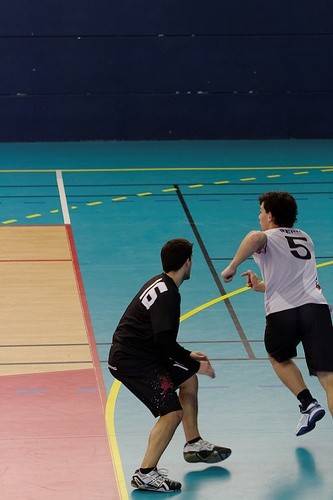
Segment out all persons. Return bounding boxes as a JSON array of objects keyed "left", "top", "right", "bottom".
[
  {"left": 107, "top": 239, "right": 231, "bottom": 493},
  {"left": 221, "top": 192, "right": 333, "bottom": 437}
]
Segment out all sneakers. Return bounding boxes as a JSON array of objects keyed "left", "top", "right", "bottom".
[
  {"left": 295, "top": 399, "right": 326, "bottom": 436},
  {"left": 183, "top": 439, "right": 232, "bottom": 463},
  {"left": 130, "top": 467, "right": 182, "bottom": 493}
]
